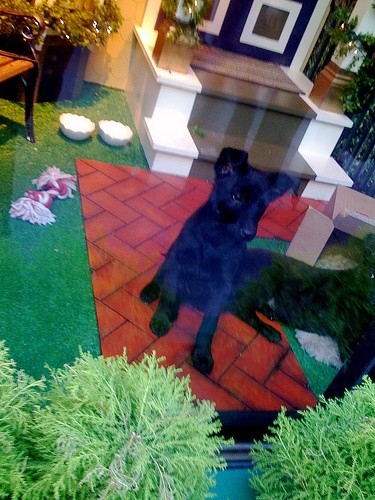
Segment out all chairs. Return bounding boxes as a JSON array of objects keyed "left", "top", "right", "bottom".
[{"left": 1, "top": 8, "right": 47, "bottom": 147}]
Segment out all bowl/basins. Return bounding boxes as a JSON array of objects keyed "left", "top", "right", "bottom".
[
  {"left": 98, "top": 120, "right": 133, "bottom": 147},
  {"left": 58, "top": 112, "right": 95, "bottom": 141}
]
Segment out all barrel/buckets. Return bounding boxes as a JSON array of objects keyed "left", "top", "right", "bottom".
[{"left": 37, "top": 44, "right": 89, "bottom": 104}]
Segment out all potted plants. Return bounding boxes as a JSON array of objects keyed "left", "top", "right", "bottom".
[
  {"left": 2, "top": 0, "right": 124, "bottom": 102},
  {"left": 152, "top": 0, "right": 208, "bottom": 74}
]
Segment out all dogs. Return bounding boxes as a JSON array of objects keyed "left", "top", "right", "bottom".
[
  {"left": 231, "top": 232, "right": 374, "bottom": 405},
  {"left": 140, "top": 147, "right": 294, "bottom": 377}
]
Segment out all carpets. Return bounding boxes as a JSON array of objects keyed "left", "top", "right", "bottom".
[{"left": 74, "top": 156, "right": 329, "bottom": 412}]
